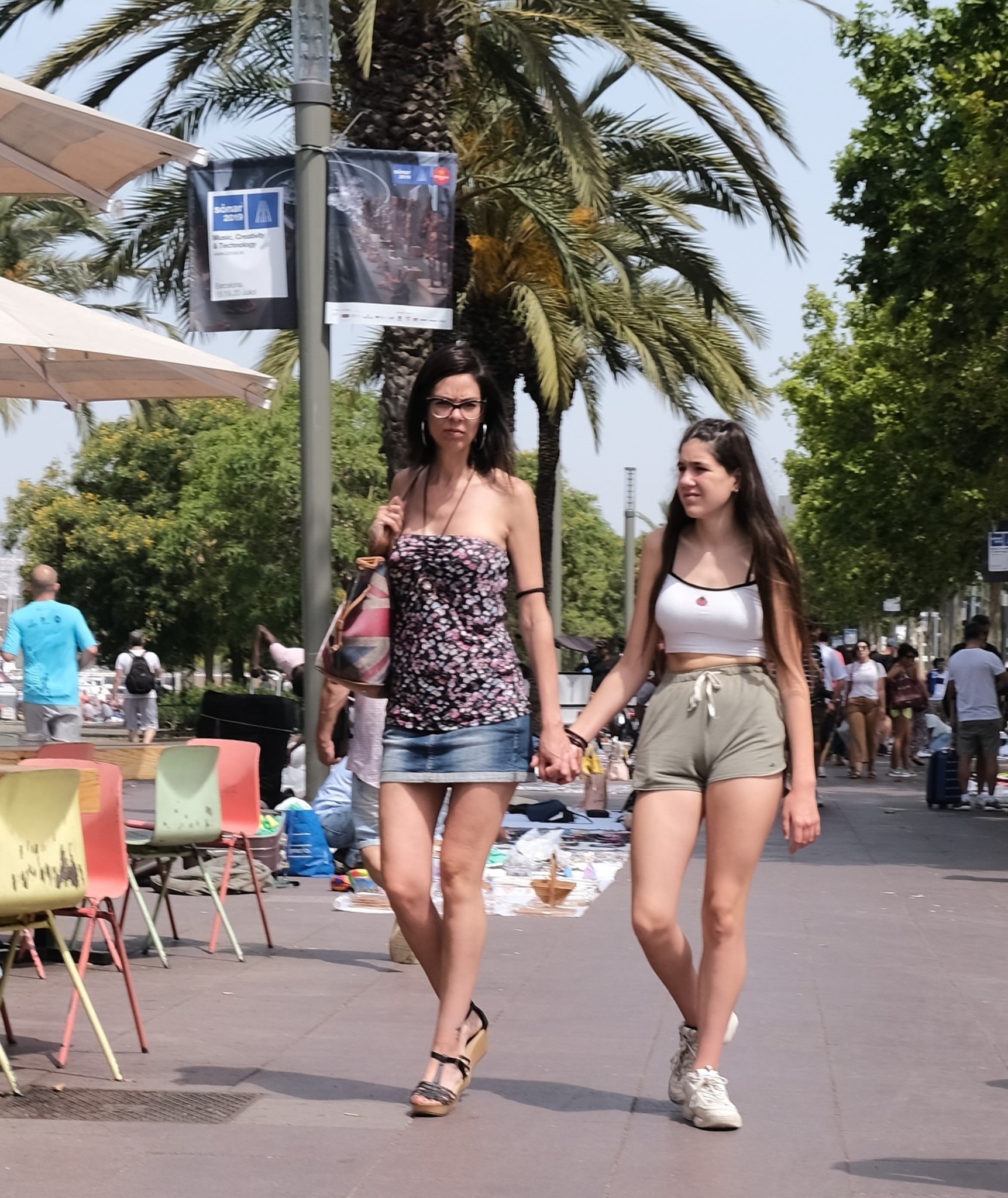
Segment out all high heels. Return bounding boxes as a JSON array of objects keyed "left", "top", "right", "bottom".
[
  {"left": 409, "top": 1050, "right": 470, "bottom": 1119},
  {"left": 453, "top": 999, "right": 489, "bottom": 1069}
]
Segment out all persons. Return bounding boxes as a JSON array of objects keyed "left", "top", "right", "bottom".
[
  {"left": 802, "top": 614, "right": 1008, "bottom": 810},
  {"left": 112, "top": 630, "right": 163, "bottom": 744},
  {"left": 367, "top": 345, "right": 583, "bottom": 1118},
  {"left": 0, "top": 564, "right": 98, "bottom": 744},
  {"left": 250, "top": 622, "right": 366, "bottom": 871},
  {"left": 314, "top": 550, "right": 423, "bottom": 965},
  {"left": 533, "top": 418, "right": 820, "bottom": 1131}
]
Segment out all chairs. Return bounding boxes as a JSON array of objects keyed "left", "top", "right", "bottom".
[{"left": 0, "top": 734, "right": 275, "bottom": 1101}]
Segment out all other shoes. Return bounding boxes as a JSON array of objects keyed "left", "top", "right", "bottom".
[
  {"left": 888, "top": 767, "right": 917, "bottom": 777},
  {"left": 816, "top": 766, "right": 826, "bottom": 777},
  {"left": 851, "top": 770, "right": 862, "bottom": 778},
  {"left": 868, "top": 768, "right": 877, "bottom": 778},
  {"left": 984, "top": 801, "right": 1002, "bottom": 811},
  {"left": 952, "top": 801, "right": 970, "bottom": 810}
]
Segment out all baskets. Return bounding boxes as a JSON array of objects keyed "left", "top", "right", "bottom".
[{"left": 530, "top": 851, "right": 577, "bottom": 904}]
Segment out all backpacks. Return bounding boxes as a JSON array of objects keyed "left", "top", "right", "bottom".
[{"left": 124, "top": 651, "right": 153, "bottom": 694}]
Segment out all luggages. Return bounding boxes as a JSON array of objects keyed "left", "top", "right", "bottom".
[{"left": 926, "top": 746, "right": 961, "bottom": 807}]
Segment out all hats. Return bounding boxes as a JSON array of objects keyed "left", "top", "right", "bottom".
[
  {"left": 887, "top": 638, "right": 898, "bottom": 648},
  {"left": 595, "top": 640, "right": 609, "bottom": 647}
]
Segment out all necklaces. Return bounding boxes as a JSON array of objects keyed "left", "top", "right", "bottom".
[{"left": 416, "top": 462, "right": 476, "bottom": 600}]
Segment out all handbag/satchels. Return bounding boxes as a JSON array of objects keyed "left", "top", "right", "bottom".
[
  {"left": 313, "top": 555, "right": 390, "bottom": 700},
  {"left": 278, "top": 810, "right": 336, "bottom": 877},
  {"left": 891, "top": 677, "right": 928, "bottom": 713}
]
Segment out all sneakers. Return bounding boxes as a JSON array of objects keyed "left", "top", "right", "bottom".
[
  {"left": 681, "top": 1065, "right": 741, "bottom": 1130},
  {"left": 668, "top": 1010, "right": 740, "bottom": 1106}
]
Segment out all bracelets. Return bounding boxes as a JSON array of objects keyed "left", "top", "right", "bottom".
[
  {"left": 515, "top": 588, "right": 546, "bottom": 601},
  {"left": 565, "top": 728, "right": 588, "bottom": 749}
]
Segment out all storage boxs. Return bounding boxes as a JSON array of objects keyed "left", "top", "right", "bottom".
[{"left": 220, "top": 806, "right": 288, "bottom": 872}]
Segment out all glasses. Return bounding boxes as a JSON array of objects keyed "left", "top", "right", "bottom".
[
  {"left": 907, "top": 655, "right": 915, "bottom": 658},
  {"left": 857, "top": 646, "right": 867, "bottom": 650},
  {"left": 426, "top": 395, "right": 488, "bottom": 420}
]
